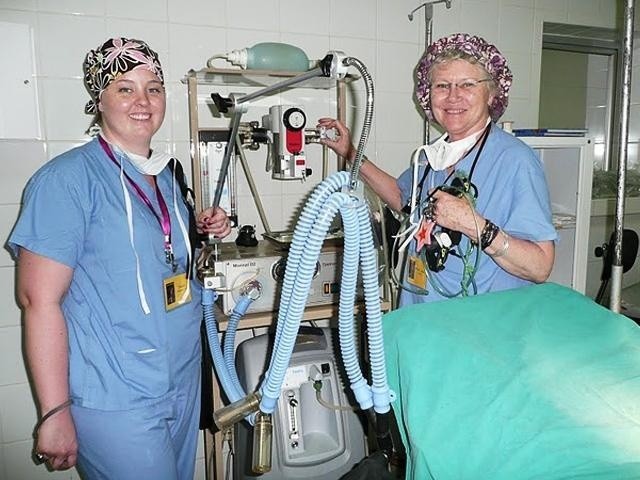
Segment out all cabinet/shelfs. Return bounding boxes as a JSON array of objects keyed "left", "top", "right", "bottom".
[
  {"left": 180, "top": 54, "right": 398, "bottom": 478},
  {"left": 516, "top": 132, "right": 597, "bottom": 291}
]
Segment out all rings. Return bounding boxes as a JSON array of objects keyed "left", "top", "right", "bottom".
[{"left": 37, "top": 453, "right": 44, "bottom": 460}]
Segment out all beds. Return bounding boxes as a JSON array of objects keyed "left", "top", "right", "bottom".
[{"left": 378, "top": 276, "right": 640, "bottom": 479}]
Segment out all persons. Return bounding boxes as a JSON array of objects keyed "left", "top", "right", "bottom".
[
  {"left": 315, "top": 33, "right": 560, "bottom": 306},
  {"left": 6, "top": 36, "right": 231, "bottom": 479}
]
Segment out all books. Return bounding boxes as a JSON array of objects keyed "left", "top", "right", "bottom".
[{"left": 512, "top": 128, "right": 589, "bottom": 138}]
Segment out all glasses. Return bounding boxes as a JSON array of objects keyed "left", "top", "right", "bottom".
[{"left": 430, "top": 78, "right": 492, "bottom": 95}]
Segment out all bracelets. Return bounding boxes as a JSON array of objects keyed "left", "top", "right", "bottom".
[
  {"left": 477, "top": 218, "right": 500, "bottom": 251},
  {"left": 358, "top": 156, "right": 367, "bottom": 172}
]
